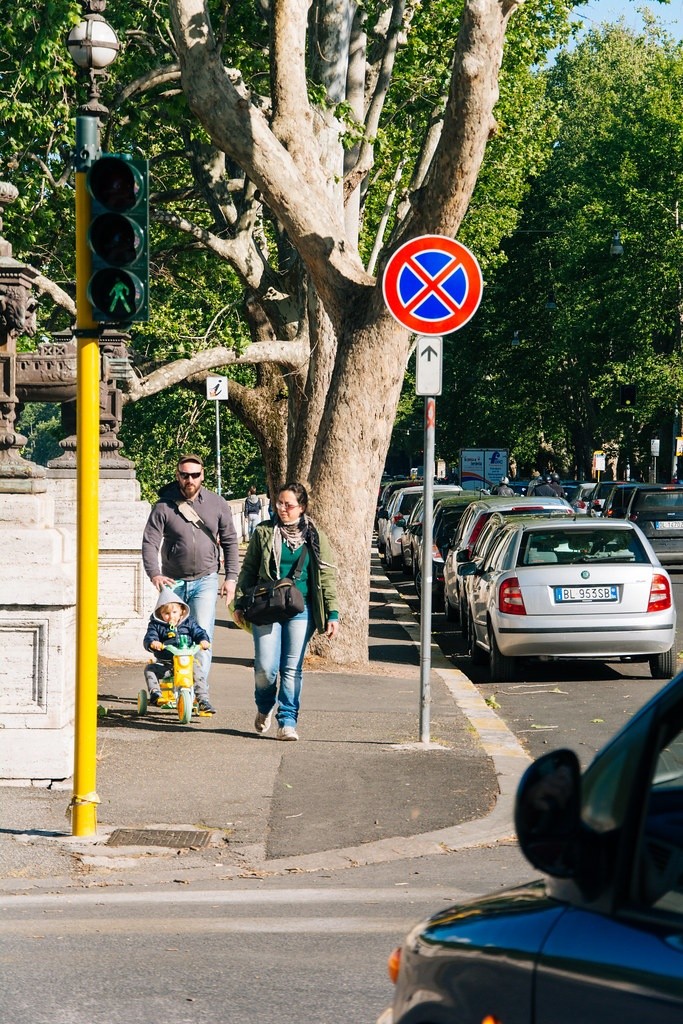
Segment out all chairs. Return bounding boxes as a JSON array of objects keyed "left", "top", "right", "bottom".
[{"left": 528, "top": 551, "right": 557, "bottom": 563}]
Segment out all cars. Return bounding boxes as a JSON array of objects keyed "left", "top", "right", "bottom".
[
  {"left": 614, "top": 483, "right": 683, "bottom": 566},
  {"left": 457, "top": 516, "right": 678, "bottom": 683},
  {"left": 387, "top": 663, "right": 682, "bottom": 1023},
  {"left": 374, "top": 471, "right": 683, "bottom": 613},
  {"left": 455, "top": 511, "right": 636, "bottom": 657},
  {"left": 436, "top": 497, "right": 579, "bottom": 640}
]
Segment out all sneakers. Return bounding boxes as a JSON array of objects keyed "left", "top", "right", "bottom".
[
  {"left": 150, "top": 691, "right": 162, "bottom": 703},
  {"left": 198, "top": 701, "right": 216, "bottom": 714},
  {"left": 276, "top": 725, "right": 297, "bottom": 742},
  {"left": 255, "top": 705, "right": 274, "bottom": 732}
]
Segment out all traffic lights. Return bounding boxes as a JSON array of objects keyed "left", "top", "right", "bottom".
[{"left": 85, "top": 155, "right": 149, "bottom": 324}]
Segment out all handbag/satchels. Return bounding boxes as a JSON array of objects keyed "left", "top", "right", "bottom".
[{"left": 243, "top": 577, "right": 304, "bottom": 627}]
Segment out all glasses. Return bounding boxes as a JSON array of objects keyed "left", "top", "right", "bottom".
[
  {"left": 276, "top": 501, "right": 301, "bottom": 510},
  {"left": 177, "top": 470, "right": 201, "bottom": 478}
]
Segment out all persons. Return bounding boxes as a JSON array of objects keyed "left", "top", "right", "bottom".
[
  {"left": 141, "top": 453, "right": 239, "bottom": 714},
  {"left": 143, "top": 588, "right": 216, "bottom": 713},
  {"left": 234, "top": 483, "right": 338, "bottom": 741},
  {"left": 244, "top": 482, "right": 273, "bottom": 541},
  {"left": 491, "top": 471, "right": 566, "bottom": 499}
]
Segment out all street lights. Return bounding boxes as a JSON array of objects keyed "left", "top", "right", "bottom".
[{"left": 59, "top": 0, "right": 124, "bottom": 835}]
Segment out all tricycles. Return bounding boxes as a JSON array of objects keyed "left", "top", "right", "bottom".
[{"left": 137, "top": 643, "right": 213, "bottom": 724}]
